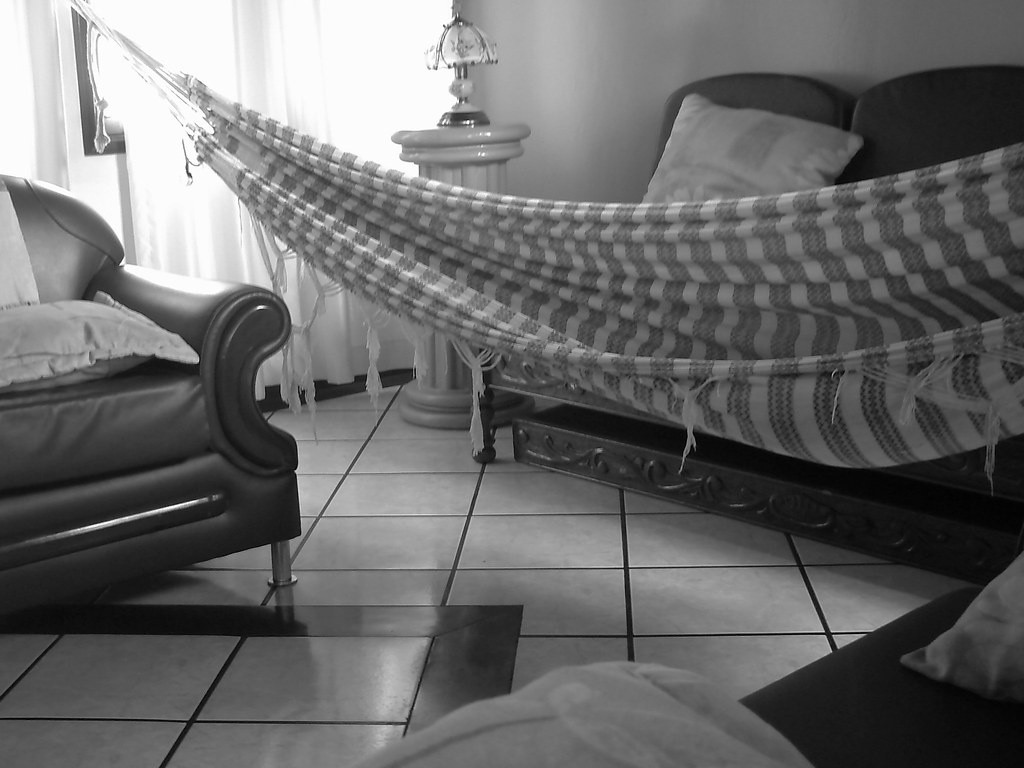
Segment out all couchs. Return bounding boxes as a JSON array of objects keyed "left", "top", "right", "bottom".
[
  {"left": 468, "top": 59, "right": 1024, "bottom": 582},
  {"left": 0, "top": 174, "right": 298, "bottom": 613}
]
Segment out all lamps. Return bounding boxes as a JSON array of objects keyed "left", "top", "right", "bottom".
[{"left": 423, "top": 0, "right": 501, "bottom": 128}]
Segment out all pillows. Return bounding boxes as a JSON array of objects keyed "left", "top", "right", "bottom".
[
  {"left": 1, "top": 292, "right": 200, "bottom": 390},
  {"left": 636, "top": 91, "right": 862, "bottom": 204}
]
